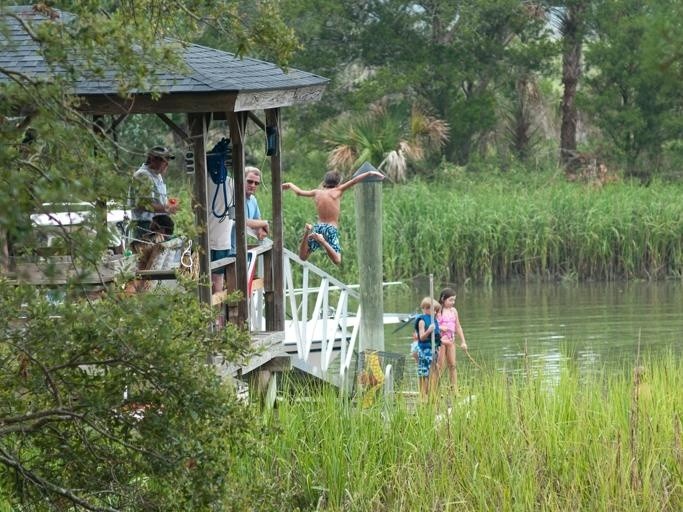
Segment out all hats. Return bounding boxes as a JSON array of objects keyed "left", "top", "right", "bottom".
[{"left": 146, "top": 146, "right": 176, "bottom": 160}]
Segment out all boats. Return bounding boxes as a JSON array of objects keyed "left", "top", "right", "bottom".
[{"left": 1, "top": 199, "right": 421, "bottom": 379}]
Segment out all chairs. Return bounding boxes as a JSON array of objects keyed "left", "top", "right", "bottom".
[{"left": 134, "top": 236, "right": 192, "bottom": 295}]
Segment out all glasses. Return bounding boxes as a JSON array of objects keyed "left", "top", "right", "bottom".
[{"left": 247, "top": 180, "right": 260, "bottom": 185}]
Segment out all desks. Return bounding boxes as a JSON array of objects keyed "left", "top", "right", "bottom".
[{"left": 2, "top": 253, "right": 135, "bottom": 300}]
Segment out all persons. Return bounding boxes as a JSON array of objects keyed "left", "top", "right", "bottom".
[
  {"left": 88, "top": 215, "right": 174, "bottom": 300},
  {"left": 411, "top": 288, "right": 467, "bottom": 403},
  {"left": 126, "top": 146, "right": 176, "bottom": 238},
  {"left": 190, "top": 173, "right": 235, "bottom": 320},
  {"left": 228, "top": 165, "right": 269, "bottom": 254},
  {"left": 282, "top": 171, "right": 383, "bottom": 264}
]
